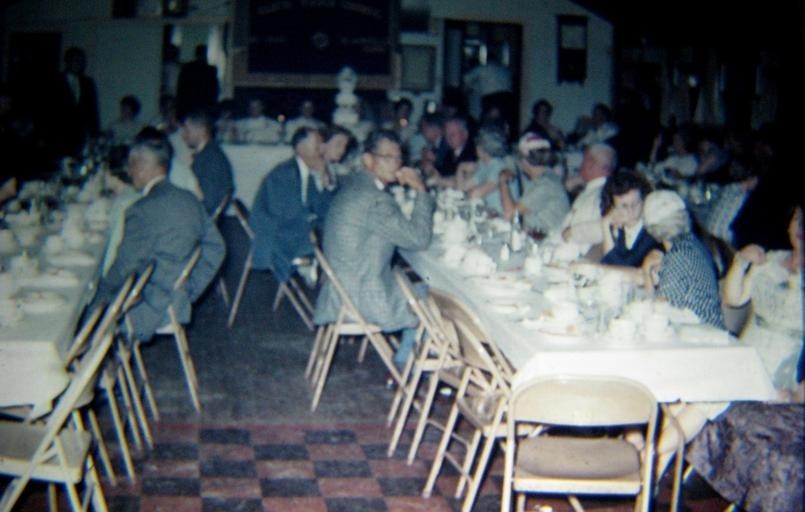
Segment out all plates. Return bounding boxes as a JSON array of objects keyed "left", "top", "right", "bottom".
[
  {"left": 48, "top": 252, "right": 95, "bottom": 270},
  {"left": 17, "top": 295, "right": 63, "bottom": 315},
  {"left": 489, "top": 302, "right": 531, "bottom": 321},
  {"left": 656, "top": 307, "right": 699, "bottom": 326},
  {"left": 37, "top": 274, "right": 82, "bottom": 288},
  {"left": 482, "top": 279, "right": 531, "bottom": 301}
]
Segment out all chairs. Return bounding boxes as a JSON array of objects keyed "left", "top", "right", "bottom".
[
  {"left": 128, "top": 244, "right": 205, "bottom": 420},
  {"left": 78, "top": 276, "right": 142, "bottom": 490},
  {"left": 422, "top": 320, "right": 584, "bottom": 512},
  {"left": 100, "top": 261, "right": 155, "bottom": 449},
  {"left": 308, "top": 247, "right": 423, "bottom": 413},
  {"left": 229, "top": 201, "right": 316, "bottom": 333},
  {"left": 408, "top": 290, "right": 512, "bottom": 469},
  {"left": 385, "top": 272, "right": 456, "bottom": 460}
]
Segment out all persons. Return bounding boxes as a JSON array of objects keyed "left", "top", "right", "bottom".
[
  {"left": 317, "top": 127, "right": 353, "bottom": 193},
  {"left": 599, "top": 168, "right": 668, "bottom": 268},
  {"left": 230, "top": 100, "right": 282, "bottom": 145},
  {"left": 177, "top": 45, "right": 221, "bottom": 127},
  {"left": 638, "top": 190, "right": 727, "bottom": 327},
  {"left": 312, "top": 128, "right": 437, "bottom": 391},
  {"left": 545, "top": 142, "right": 615, "bottom": 265},
  {"left": 105, "top": 126, "right": 202, "bottom": 276},
  {"left": 465, "top": 61, "right": 513, "bottom": 129},
  {"left": 405, "top": 113, "right": 445, "bottom": 168},
  {"left": 422, "top": 119, "right": 479, "bottom": 178},
  {"left": 248, "top": 126, "right": 333, "bottom": 290},
  {"left": 80, "top": 126, "right": 227, "bottom": 391},
  {"left": 527, "top": 99, "right": 565, "bottom": 148},
  {"left": 429, "top": 128, "right": 519, "bottom": 223},
  {"left": 500, "top": 130, "right": 570, "bottom": 240},
  {"left": 180, "top": 109, "right": 236, "bottom": 225},
  {"left": 385, "top": 97, "right": 420, "bottom": 144},
  {"left": 53, "top": 44, "right": 101, "bottom": 169},
  {"left": 284, "top": 100, "right": 324, "bottom": 142},
  {"left": 723, "top": 198, "right": 805, "bottom": 406},
  {"left": 105, "top": 95, "right": 144, "bottom": 147},
  {"left": 580, "top": 101, "right": 619, "bottom": 151}
]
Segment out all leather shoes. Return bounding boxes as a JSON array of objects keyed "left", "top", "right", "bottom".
[
  {"left": 417, "top": 382, "right": 454, "bottom": 405},
  {"left": 383, "top": 369, "right": 399, "bottom": 389}
]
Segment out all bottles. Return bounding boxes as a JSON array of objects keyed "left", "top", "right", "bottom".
[{"left": 510, "top": 208, "right": 523, "bottom": 252}]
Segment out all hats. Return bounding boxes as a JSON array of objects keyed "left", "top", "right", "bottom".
[
  {"left": 644, "top": 191, "right": 685, "bottom": 226},
  {"left": 478, "top": 125, "right": 507, "bottom": 154}
]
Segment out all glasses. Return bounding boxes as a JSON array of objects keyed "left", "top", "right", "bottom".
[{"left": 370, "top": 153, "right": 402, "bottom": 162}]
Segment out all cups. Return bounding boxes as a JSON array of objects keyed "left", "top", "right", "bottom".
[
  {"left": 10, "top": 250, "right": 40, "bottom": 285},
  {"left": 0, "top": 299, "right": 24, "bottom": 329},
  {"left": 610, "top": 318, "right": 636, "bottom": 353},
  {"left": 642, "top": 315, "right": 669, "bottom": 344}
]
